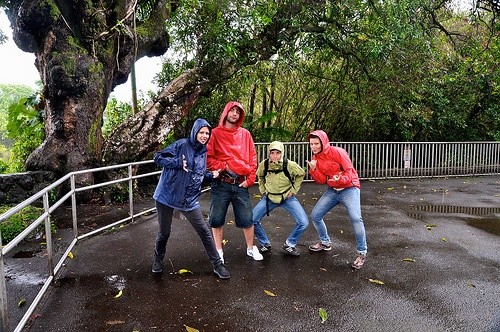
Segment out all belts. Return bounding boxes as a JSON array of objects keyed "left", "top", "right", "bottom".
[{"left": 218, "top": 176, "right": 245, "bottom": 184}]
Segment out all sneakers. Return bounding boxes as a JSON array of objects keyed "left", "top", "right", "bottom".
[
  {"left": 218, "top": 252, "right": 224, "bottom": 264},
  {"left": 308, "top": 241, "right": 331, "bottom": 251},
  {"left": 213, "top": 264, "right": 230, "bottom": 278},
  {"left": 256, "top": 244, "right": 271, "bottom": 253},
  {"left": 352, "top": 255, "right": 365, "bottom": 269},
  {"left": 247, "top": 246, "right": 263, "bottom": 260},
  {"left": 152, "top": 256, "right": 163, "bottom": 272},
  {"left": 281, "top": 243, "right": 300, "bottom": 256}
]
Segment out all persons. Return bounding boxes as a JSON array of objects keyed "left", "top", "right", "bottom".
[
  {"left": 250, "top": 141, "right": 308, "bottom": 257},
  {"left": 304, "top": 130, "right": 368, "bottom": 269},
  {"left": 151, "top": 118, "right": 231, "bottom": 279},
  {"left": 205, "top": 101, "right": 264, "bottom": 265}
]
